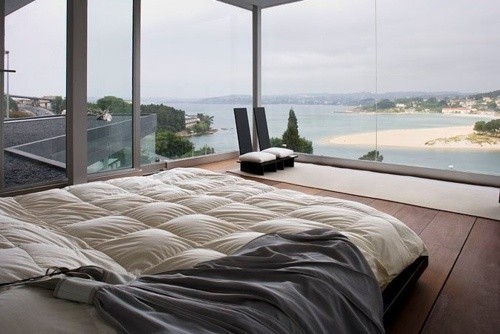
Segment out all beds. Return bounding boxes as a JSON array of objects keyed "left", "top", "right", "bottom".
[{"left": 0, "top": 164, "right": 430, "bottom": 334}]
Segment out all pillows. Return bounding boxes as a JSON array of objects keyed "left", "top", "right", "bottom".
[
  {"left": 236, "top": 151, "right": 276, "bottom": 164},
  {"left": 261, "top": 147, "right": 293, "bottom": 159}
]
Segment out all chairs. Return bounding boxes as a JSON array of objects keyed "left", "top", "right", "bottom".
[
  {"left": 232, "top": 108, "right": 277, "bottom": 175},
  {"left": 253, "top": 107, "right": 299, "bottom": 171}
]
